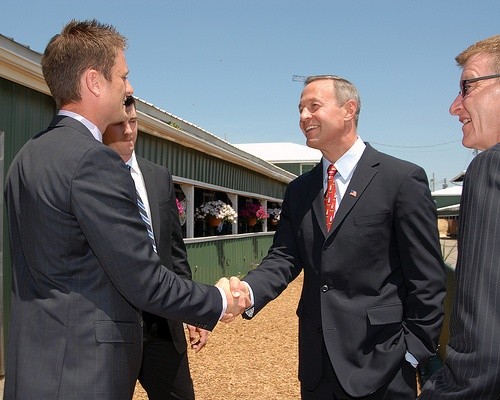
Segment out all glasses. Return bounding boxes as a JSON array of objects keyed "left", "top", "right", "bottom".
[{"left": 459, "top": 74, "right": 500, "bottom": 98}]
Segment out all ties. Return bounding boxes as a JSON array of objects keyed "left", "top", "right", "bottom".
[
  {"left": 125, "top": 164, "right": 157, "bottom": 254},
  {"left": 323, "top": 164, "right": 338, "bottom": 232}
]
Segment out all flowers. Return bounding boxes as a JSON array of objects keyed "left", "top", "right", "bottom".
[
  {"left": 176, "top": 198, "right": 186, "bottom": 226},
  {"left": 194, "top": 200, "right": 239, "bottom": 225},
  {"left": 241, "top": 200, "right": 282, "bottom": 224}
]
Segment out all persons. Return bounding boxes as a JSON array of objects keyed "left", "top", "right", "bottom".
[
  {"left": 415, "top": 37, "right": 500, "bottom": 400},
  {"left": 6, "top": 19, "right": 252, "bottom": 400},
  {"left": 102, "top": 96, "right": 208, "bottom": 400},
  {"left": 219, "top": 75, "right": 446, "bottom": 400}
]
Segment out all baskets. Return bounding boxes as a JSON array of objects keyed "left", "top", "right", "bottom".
[
  {"left": 270, "top": 218, "right": 278, "bottom": 225},
  {"left": 179, "top": 216, "right": 186, "bottom": 226},
  {"left": 208, "top": 214, "right": 220, "bottom": 227},
  {"left": 247, "top": 215, "right": 257, "bottom": 227}
]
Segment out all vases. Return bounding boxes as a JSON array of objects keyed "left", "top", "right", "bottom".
[
  {"left": 210, "top": 217, "right": 220, "bottom": 226},
  {"left": 247, "top": 216, "right": 257, "bottom": 226}
]
